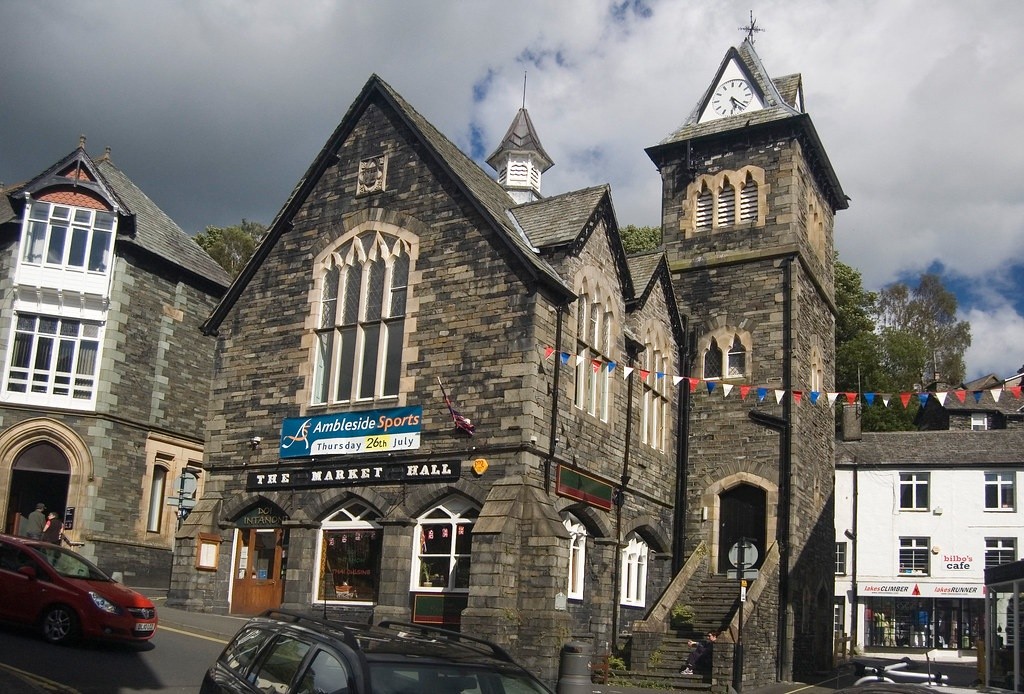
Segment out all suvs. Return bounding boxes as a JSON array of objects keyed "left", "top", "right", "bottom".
[{"left": 199, "top": 609, "right": 558, "bottom": 694}]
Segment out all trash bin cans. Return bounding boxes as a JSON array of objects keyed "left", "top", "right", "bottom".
[{"left": 556, "top": 640, "right": 595, "bottom": 694}]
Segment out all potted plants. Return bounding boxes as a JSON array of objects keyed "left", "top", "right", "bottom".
[{"left": 421, "top": 562, "right": 439, "bottom": 587}]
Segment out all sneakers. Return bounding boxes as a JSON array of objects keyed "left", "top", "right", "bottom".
[{"left": 680, "top": 666, "right": 694, "bottom": 675}]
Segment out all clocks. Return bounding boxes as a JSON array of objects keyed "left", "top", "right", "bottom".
[{"left": 712, "top": 79, "right": 753, "bottom": 117}]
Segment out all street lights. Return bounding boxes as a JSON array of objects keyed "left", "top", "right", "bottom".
[
  {"left": 844, "top": 527, "right": 857, "bottom": 657},
  {"left": 748, "top": 406, "right": 792, "bottom": 684},
  {"left": 177, "top": 466, "right": 203, "bottom": 531}
]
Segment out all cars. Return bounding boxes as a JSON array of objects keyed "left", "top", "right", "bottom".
[
  {"left": 831, "top": 678, "right": 1024, "bottom": 694},
  {"left": 0, "top": 534, "right": 159, "bottom": 646}
]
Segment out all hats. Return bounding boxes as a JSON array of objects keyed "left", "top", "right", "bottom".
[{"left": 36, "top": 502, "right": 47, "bottom": 509}]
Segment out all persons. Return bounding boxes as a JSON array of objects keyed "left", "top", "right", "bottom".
[
  {"left": 26, "top": 504, "right": 47, "bottom": 540},
  {"left": 679, "top": 632, "right": 718, "bottom": 675},
  {"left": 41, "top": 512, "right": 65, "bottom": 566}
]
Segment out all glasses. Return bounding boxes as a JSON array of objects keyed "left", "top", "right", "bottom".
[{"left": 705, "top": 635, "right": 712, "bottom": 637}]
